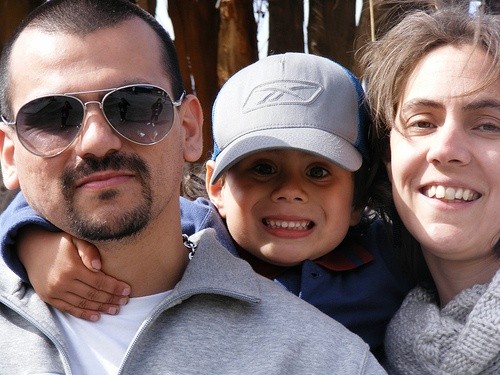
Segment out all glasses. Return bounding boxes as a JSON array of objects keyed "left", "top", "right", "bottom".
[{"left": 2, "top": 83, "right": 187, "bottom": 160}]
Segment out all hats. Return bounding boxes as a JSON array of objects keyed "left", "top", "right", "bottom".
[{"left": 211, "top": 50, "right": 370, "bottom": 184}]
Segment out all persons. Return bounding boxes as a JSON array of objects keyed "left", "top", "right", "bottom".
[
  {"left": 147, "top": 98, "right": 163, "bottom": 127},
  {"left": 59, "top": 101, "right": 73, "bottom": 128},
  {"left": 118, "top": 97, "right": 131, "bottom": 121},
  {"left": 357, "top": 9, "right": 500, "bottom": 375},
  {"left": 0, "top": 1, "right": 387, "bottom": 375},
  {"left": 1, "top": 52, "right": 432, "bottom": 363}
]
